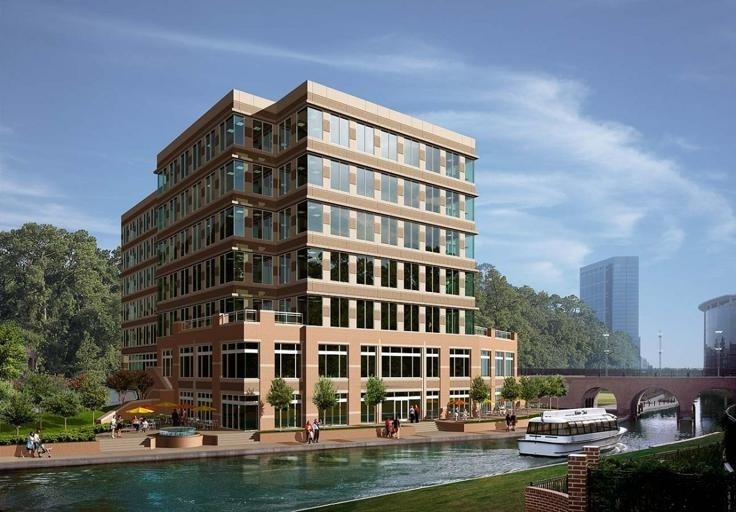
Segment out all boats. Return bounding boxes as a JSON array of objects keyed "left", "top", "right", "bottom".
[{"left": 517, "top": 407, "right": 628, "bottom": 457}]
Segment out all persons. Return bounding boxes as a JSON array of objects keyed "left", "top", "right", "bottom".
[
  {"left": 409, "top": 405, "right": 415, "bottom": 423},
  {"left": 111, "top": 414, "right": 148, "bottom": 438},
  {"left": 415, "top": 404, "right": 420, "bottom": 422},
  {"left": 305, "top": 421, "right": 313, "bottom": 441},
  {"left": 385, "top": 416, "right": 401, "bottom": 440},
  {"left": 172, "top": 408, "right": 188, "bottom": 426},
  {"left": 506, "top": 414, "right": 511, "bottom": 432},
  {"left": 511, "top": 412, "right": 516, "bottom": 431},
  {"left": 308, "top": 429, "right": 315, "bottom": 444},
  {"left": 314, "top": 419, "right": 320, "bottom": 443},
  {"left": 26, "top": 429, "right": 45, "bottom": 457}
]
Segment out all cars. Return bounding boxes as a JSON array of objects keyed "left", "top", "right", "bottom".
[{"left": 188, "top": 418, "right": 212, "bottom": 430}]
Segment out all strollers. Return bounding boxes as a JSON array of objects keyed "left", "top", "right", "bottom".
[{"left": 34, "top": 439, "right": 51, "bottom": 458}]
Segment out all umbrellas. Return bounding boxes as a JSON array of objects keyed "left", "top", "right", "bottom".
[
  {"left": 192, "top": 404, "right": 216, "bottom": 420},
  {"left": 154, "top": 402, "right": 177, "bottom": 414},
  {"left": 450, "top": 397, "right": 469, "bottom": 411},
  {"left": 178, "top": 403, "right": 196, "bottom": 409},
  {"left": 126, "top": 406, "right": 155, "bottom": 417},
  {"left": 477, "top": 398, "right": 498, "bottom": 414}
]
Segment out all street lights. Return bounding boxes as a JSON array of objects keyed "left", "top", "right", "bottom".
[
  {"left": 602, "top": 333, "right": 610, "bottom": 375},
  {"left": 714, "top": 331, "right": 724, "bottom": 376},
  {"left": 657, "top": 330, "right": 663, "bottom": 377}
]
